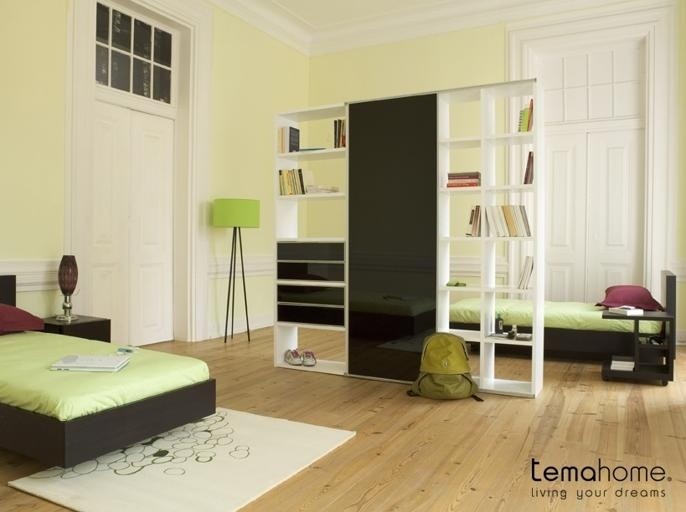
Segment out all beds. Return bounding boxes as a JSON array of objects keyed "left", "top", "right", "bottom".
[
  {"left": 449, "top": 270, "right": 677, "bottom": 364},
  {"left": 0, "top": 275, "right": 217, "bottom": 469}
]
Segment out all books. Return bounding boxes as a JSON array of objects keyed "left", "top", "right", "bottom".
[
  {"left": 607, "top": 306, "right": 644, "bottom": 317},
  {"left": 609, "top": 353, "right": 636, "bottom": 372},
  {"left": 333, "top": 118, "right": 345, "bottom": 148},
  {"left": 523, "top": 151, "right": 534, "bottom": 184},
  {"left": 277, "top": 168, "right": 338, "bottom": 194},
  {"left": 446, "top": 171, "right": 480, "bottom": 188},
  {"left": 463, "top": 203, "right": 531, "bottom": 237},
  {"left": 278, "top": 125, "right": 299, "bottom": 152},
  {"left": 47, "top": 353, "right": 131, "bottom": 373},
  {"left": 517, "top": 255, "right": 534, "bottom": 289},
  {"left": 518, "top": 97, "right": 533, "bottom": 132}
]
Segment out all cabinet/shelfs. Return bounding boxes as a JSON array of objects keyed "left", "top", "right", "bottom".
[{"left": 272, "top": 76, "right": 549, "bottom": 400}]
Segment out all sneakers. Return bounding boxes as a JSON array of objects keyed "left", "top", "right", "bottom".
[
  {"left": 285, "top": 349, "right": 302, "bottom": 365},
  {"left": 302, "top": 351, "right": 316, "bottom": 365}
]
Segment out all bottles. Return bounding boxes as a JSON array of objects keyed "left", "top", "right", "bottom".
[{"left": 495, "top": 313, "right": 503, "bottom": 334}]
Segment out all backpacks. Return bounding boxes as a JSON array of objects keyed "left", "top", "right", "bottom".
[{"left": 407, "top": 333, "right": 483, "bottom": 402}]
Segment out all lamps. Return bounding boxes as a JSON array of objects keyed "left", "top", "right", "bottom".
[
  {"left": 211, "top": 198, "right": 261, "bottom": 343},
  {"left": 56, "top": 255, "right": 78, "bottom": 321}
]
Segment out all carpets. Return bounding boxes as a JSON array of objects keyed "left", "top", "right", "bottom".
[{"left": 8, "top": 406, "right": 357, "bottom": 511}]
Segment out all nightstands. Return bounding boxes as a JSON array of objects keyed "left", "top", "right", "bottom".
[
  {"left": 42, "top": 315, "right": 111, "bottom": 343},
  {"left": 600, "top": 311, "right": 675, "bottom": 381}
]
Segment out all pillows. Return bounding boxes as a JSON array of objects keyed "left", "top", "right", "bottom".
[
  {"left": 0, "top": 302, "right": 45, "bottom": 335},
  {"left": 594, "top": 285, "right": 665, "bottom": 312}
]
